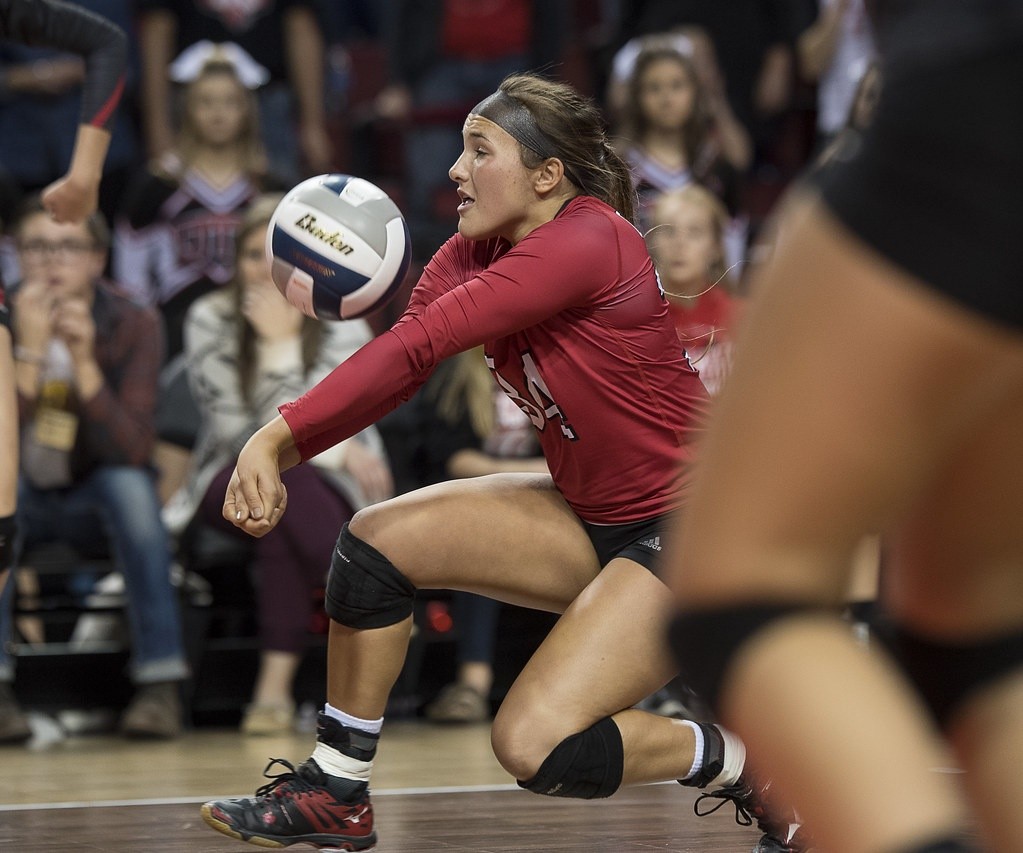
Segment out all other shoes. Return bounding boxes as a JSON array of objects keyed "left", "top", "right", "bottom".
[
  {"left": 427, "top": 682, "right": 488, "bottom": 723},
  {"left": 117, "top": 684, "right": 182, "bottom": 738},
  {"left": 0, "top": 680, "right": 32, "bottom": 743},
  {"left": 242, "top": 694, "right": 296, "bottom": 735},
  {"left": 642, "top": 689, "right": 685, "bottom": 717}
]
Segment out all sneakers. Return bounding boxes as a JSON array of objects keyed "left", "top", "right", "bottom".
[
  {"left": 693, "top": 763, "right": 815, "bottom": 852},
  {"left": 201, "top": 759, "right": 378, "bottom": 852}
]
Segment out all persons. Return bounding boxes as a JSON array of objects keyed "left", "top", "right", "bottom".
[
  {"left": 0, "top": 0, "right": 1023, "bottom": 853},
  {"left": 161, "top": 191, "right": 394, "bottom": 738}
]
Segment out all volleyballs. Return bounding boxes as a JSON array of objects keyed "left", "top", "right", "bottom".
[{"left": 265, "top": 173, "right": 416, "bottom": 326}]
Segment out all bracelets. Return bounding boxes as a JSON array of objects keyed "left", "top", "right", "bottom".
[{"left": 19, "top": 344, "right": 45, "bottom": 365}]
